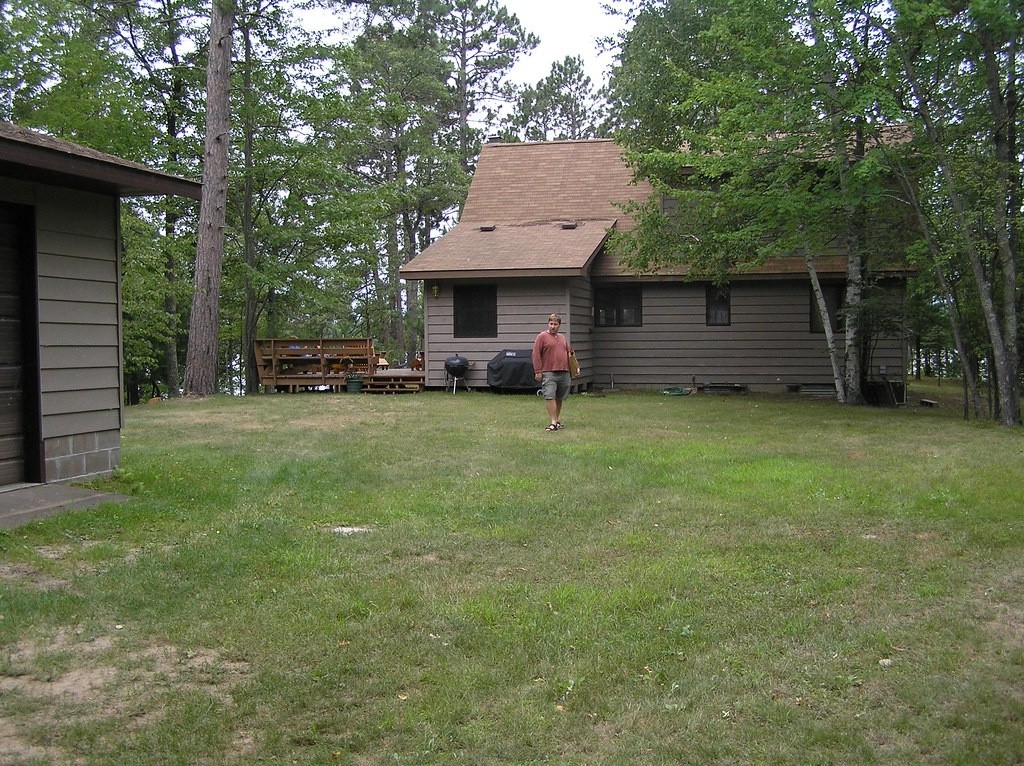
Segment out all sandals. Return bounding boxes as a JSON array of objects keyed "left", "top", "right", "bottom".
[
  {"left": 556, "top": 421, "right": 564, "bottom": 428},
  {"left": 546, "top": 424, "right": 557, "bottom": 431}
]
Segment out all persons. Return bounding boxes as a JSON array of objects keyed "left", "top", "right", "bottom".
[{"left": 532, "top": 313, "right": 581, "bottom": 431}]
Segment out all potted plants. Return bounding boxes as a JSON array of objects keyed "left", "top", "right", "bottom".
[{"left": 344, "top": 374, "right": 364, "bottom": 393}]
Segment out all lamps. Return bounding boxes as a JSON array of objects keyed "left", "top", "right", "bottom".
[{"left": 432, "top": 282, "right": 440, "bottom": 296}]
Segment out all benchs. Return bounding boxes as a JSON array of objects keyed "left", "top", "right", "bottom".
[{"left": 254, "top": 336, "right": 389, "bottom": 375}]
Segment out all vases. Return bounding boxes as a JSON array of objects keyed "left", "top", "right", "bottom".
[{"left": 786, "top": 384, "right": 801, "bottom": 392}]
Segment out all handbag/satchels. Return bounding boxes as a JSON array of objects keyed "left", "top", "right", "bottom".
[{"left": 568, "top": 349, "right": 581, "bottom": 379}]
